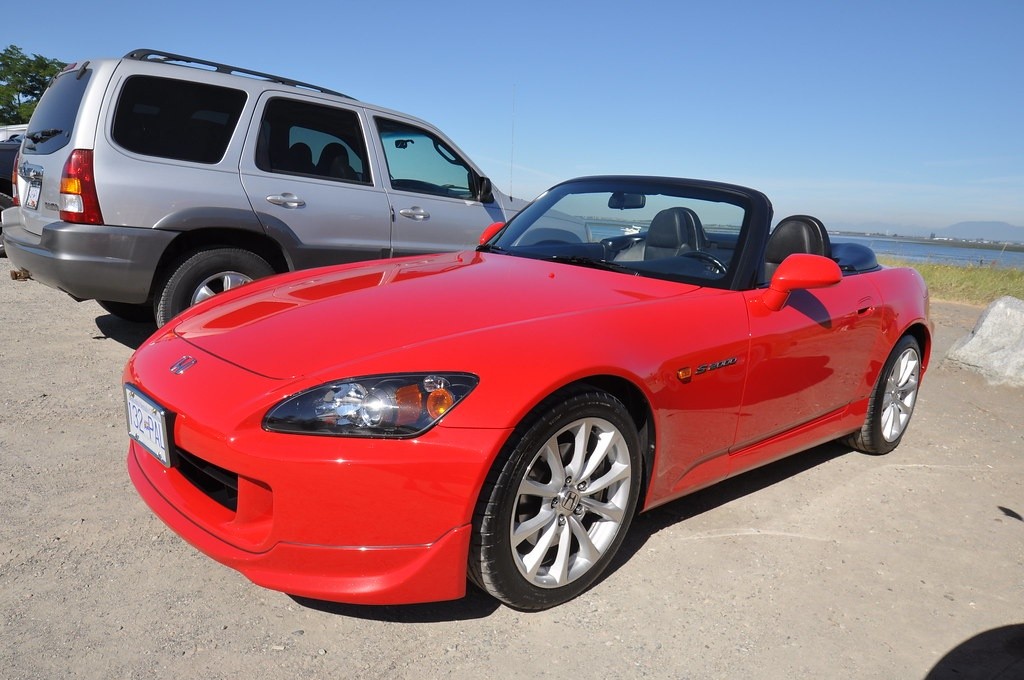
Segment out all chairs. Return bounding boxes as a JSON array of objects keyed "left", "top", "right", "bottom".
[
  {"left": 642, "top": 207, "right": 706, "bottom": 259},
  {"left": 285, "top": 142, "right": 317, "bottom": 173},
  {"left": 757, "top": 215, "right": 830, "bottom": 287},
  {"left": 313, "top": 142, "right": 360, "bottom": 181}
]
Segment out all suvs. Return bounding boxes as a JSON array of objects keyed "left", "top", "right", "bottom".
[{"left": 1, "top": 48, "right": 595, "bottom": 329}]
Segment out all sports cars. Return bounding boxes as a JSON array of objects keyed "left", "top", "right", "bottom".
[{"left": 122, "top": 174, "right": 935, "bottom": 614}]
